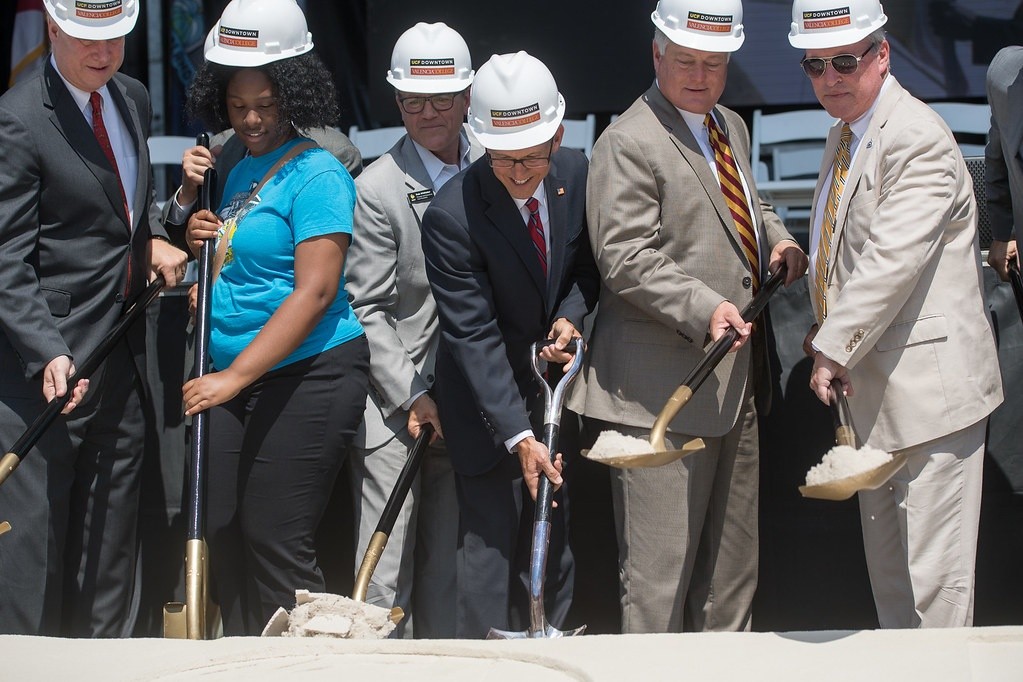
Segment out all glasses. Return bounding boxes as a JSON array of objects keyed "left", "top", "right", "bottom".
[
  {"left": 397, "top": 88, "right": 468, "bottom": 113},
  {"left": 800, "top": 42, "right": 877, "bottom": 79},
  {"left": 485, "top": 136, "right": 556, "bottom": 168}
]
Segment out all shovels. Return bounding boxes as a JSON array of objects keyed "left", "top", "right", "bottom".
[
  {"left": 798, "top": 379, "right": 907, "bottom": 502},
  {"left": 0, "top": 274, "right": 164, "bottom": 532},
  {"left": 352, "top": 421, "right": 434, "bottom": 638},
  {"left": 577, "top": 263, "right": 788, "bottom": 467},
  {"left": 484, "top": 336, "right": 589, "bottom": 639},
  {"left": 164, "top": 131, "right": 223, "bottom": 639}
]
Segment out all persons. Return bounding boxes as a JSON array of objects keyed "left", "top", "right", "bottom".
[
  {"left": 560, "top": 0, "right": 809, "bottom": 631},
  {"left": 981, "top": 45, "right": 1023, "bottom": 312},
  {"left": 434, "top": 51, "right": 603, "bottom": 640},
  {"left": 344, "top": 20, "right": 486, "bottom": 640},
  {"left": 158, "top": 1, "right": 374, "bottom": 637},
  {"left": 788, "top": 0, "right": 1006, "bottom": 628},
  {"left": 0, "top": 0, "right": 188, "bottom": 641}
]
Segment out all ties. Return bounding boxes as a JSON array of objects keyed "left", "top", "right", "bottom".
[
  {"left": 90, "top": 91, "right": 131, "bottom": 298},
  {"left": 526, "top": 196, "right": 548, "bottom": 383},
  {"left": 816, "top": 123, "right": 853, "bottom": 330},
  {"left": 704, "top": 114, "right": 759, "bottom": 331}
]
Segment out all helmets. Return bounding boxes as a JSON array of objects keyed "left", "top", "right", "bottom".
[
  {"left": 467, "top": 50, "right": 566, "bottom": 150},
  {"left": 386, "top": 22, "right": 475, "bottom": 94},
  {"left": 44, "top": 0, "right": 141, "bottom": 40},
  {"left": 204, "top": 0, "right": 314, "bottom": 67},
  {"left": 788, "top": 0, "right": 889, "bottom": 49},
  {"left": 650, "top": 0, "right": 746, "bottom": 53}
]
